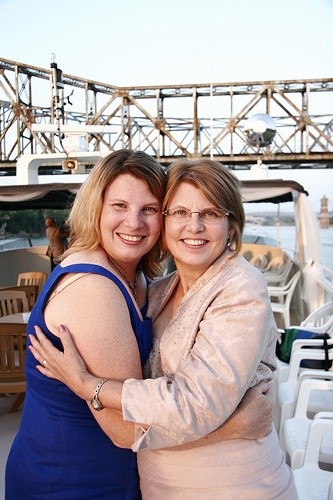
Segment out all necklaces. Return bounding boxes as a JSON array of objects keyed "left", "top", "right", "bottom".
[{"left": 107, "top": 254, "right": 138, "bottom": 305}]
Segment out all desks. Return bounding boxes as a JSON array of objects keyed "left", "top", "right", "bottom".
[{"left": 0, "top": 312, "right": 30, "bottom": 413}]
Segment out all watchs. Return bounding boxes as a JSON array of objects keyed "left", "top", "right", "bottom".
[{"left": 90, "top": 378, "right": 108, "bottom": 411}]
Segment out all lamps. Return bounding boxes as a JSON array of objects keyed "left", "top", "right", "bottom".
[{"left": 241, "top": 113, "right": 277, "bottom": 156}]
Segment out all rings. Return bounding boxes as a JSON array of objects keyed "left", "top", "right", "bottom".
[{"left": 42, "top": 360, "right": 46, "bottom": 367}]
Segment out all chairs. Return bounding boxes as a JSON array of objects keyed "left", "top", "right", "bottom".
[
  {"left": 250, "top": 255, "right": 333, "bottom": 500},
  {"left": 0, "top": 273, "right": 49, "bottom": 413}
]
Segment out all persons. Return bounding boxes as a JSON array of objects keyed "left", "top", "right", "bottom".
[
  {"left": 46, "top": 217, "right": 70, "bottom": 273},
  {"left": 5, "top": 149, "right": 273, "bottom": 500},
  {"left": 28, "top": 159, "right": 298, "bottom": 500}
]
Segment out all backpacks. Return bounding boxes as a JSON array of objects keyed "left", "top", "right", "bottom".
[{"left": 277, "top": 327, "right": 333, "bottom": 372}]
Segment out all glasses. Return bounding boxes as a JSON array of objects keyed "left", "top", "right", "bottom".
[{"left": 162, "top": 205, "right": 229, "bottom": 225}]
